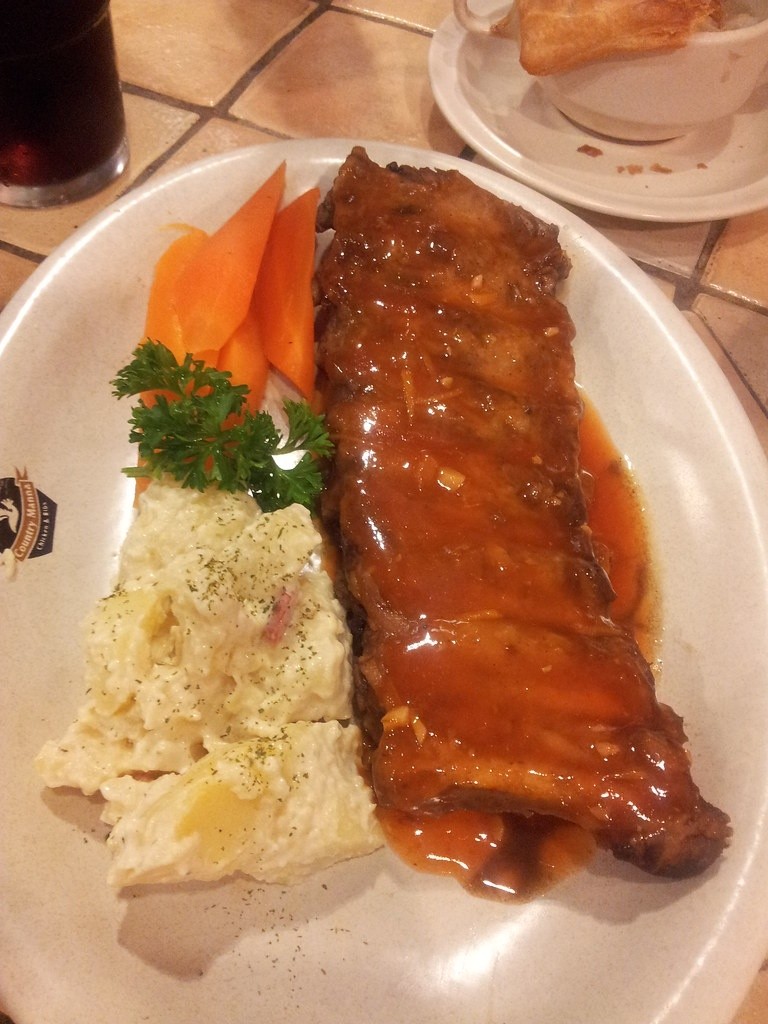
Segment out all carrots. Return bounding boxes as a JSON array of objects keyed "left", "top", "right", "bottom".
[{"left": 125, "top": 158, "right": 318, "bottom": 502}]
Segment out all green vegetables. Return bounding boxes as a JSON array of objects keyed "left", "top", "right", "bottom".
[{"left": 114, "top": 337, "right": 333, "bottom": 521}]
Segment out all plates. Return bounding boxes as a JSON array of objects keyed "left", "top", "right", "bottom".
[
  {"left": 1, "top": 135, "right": 768, "bottom": 1024},
  {"left": 428, "top": 0, "right": 768, "bottom": 223}
]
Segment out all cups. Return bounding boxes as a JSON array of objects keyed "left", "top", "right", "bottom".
[
  {"left": 455, "top": 0, "right": 768, "bottom": 143},
  {"left": 0, "top": 0, "right": 128, "bottom": 208}
]
